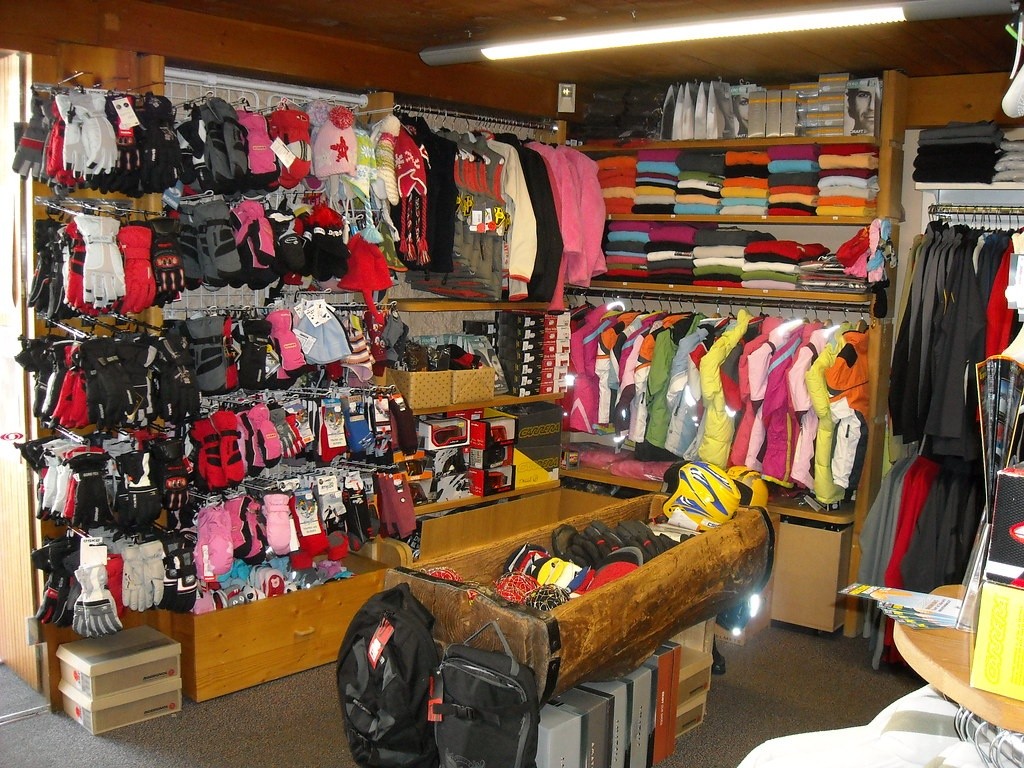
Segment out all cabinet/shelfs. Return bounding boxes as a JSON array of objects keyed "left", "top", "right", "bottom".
[
  {"left": 555, "top": 71, "right": 914, "bottom": 639},
  {"left": 133, "top": 55, "right": 569, "bottom": 567}
]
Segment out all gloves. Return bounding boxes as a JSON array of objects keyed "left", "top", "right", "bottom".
[
  {"left": 12, "top": 85, "right": 311, "bottom": 201},
  {"left": 364, "top": 311, "right": 387, "bottom": 361},
  {"left": 14, "top": 326, "right": 200, "bottom": 432},
  {"left": 169, "top": 200, "right": 352, "bottom": 292},
  {"left": 175, "top": 397, "right": 307, "bottom": 492},
  {"left": 552, "top": 519, "right": 696, "bottom": 569},
  {"left": 31, "top": 535, "right": 197, "bottom": 639},
  {"left": 163, "top": 310, "right": 308, "bottom": 397},
  {"left": 27, "top": 215, "right": 185, "bottom": 321},
  {"left": 20, "top": 430, "right": 188, "bottom": 532},
  {"left": 194, "top": 494, "right": 297, "bottom": 616}
]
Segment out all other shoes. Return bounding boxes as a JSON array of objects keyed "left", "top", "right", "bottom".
[{"left": 711, "top": 634, "right": 725, "bottom": 674}]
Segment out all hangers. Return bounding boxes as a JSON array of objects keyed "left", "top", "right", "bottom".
[
  {"left": 942, "top": 692, "right": 1024, "bottom": 768},
  {"left": 925, "top": 203, "right": 1024, "bottom": 239},
  {"left": 561, "top": 285, "right": 874, "bottom": 333},
  {"left": 391, "top": 103, "right": 562, "bottom": 151}
]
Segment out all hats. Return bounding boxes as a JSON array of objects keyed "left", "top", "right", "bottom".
[
  {"left": 306, "top": 100, "right": 431, "bottom": 322},
  {"left": 291, "top": 560, "right": 342, "bottom": 589},
  {"left": 292, "top": 308, "right": 376, "bottom": 397}
]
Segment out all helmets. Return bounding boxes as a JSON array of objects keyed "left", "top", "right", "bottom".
[
  {"left": 725, "top": 466, "right": 769, "bottom": 506},
  {"left": 660, "top": 460, "right": 741, "bottom": 532}
]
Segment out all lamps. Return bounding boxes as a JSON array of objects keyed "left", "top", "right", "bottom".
[{"left": 417, "top": 0, "right": 1020, "bottom": 67}]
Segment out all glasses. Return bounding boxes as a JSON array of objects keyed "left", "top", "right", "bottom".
[
  {"left": 491, "top": 425, "right": 508, "bottom": 443},
  {"left": 487, "top": 472, "right": 504, "bottom": 489},
  {"left": 431, "top": 425, "right": 463, "bottom": 447}
]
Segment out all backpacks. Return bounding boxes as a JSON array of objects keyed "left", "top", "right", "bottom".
[
  {"left": 427, "top": 620, "right": 540, "bottom": 768},
  {"left": 336, "top": 582, "right": 438, "bottom": 768}
]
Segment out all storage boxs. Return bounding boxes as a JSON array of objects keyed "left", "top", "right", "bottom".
[
  {"left": 160, "top": 550, "right": 393, "bottom": 704},
  {"left": 58, "top": 677, "right": 183, "bottom": 736},
  {"left": 770, "top": 521, "right": 854, "bottom": 633},
  {"left": 748, "top": 73, "right": 883, "bottom": 140},
  {"left": 535, "top": 641, "right": 715, "bottom": 768},
  {"left": 464, "top": 310, "right": 571, "bottom": 397},
  {"left": 54, "top": 624, "right": 182, "bottom": 699},
  {"left": 984, "top": 464, "right": 1024, "bottom": 592},
  {"left": 449, "top": 363, "right": 494, "bottom": 404},
  {"left": 419, "top": 490, "right": 561, "bottom": 561},
  {"left": 393, "top": 401, "right": 563, "bottom": 507},
  {"left": 968, "top": 582, "right": 1024, "bottom": 702},
  {"left": 386, "top": 366, "right": 450, "bottom": 408},
  {"left": 559, "top": 487, "right": 622, "bottom": 520}
]
